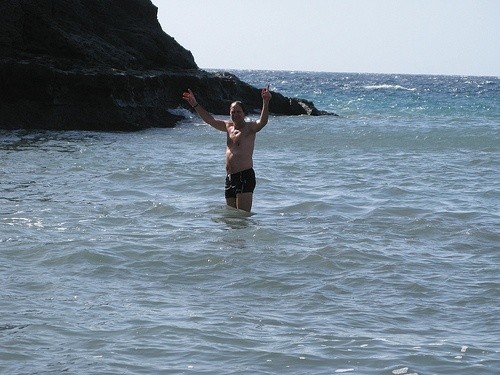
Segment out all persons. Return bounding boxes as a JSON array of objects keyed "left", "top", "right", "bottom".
[{"left": 182, "top": 84, "right": 273, "bottom": 212}]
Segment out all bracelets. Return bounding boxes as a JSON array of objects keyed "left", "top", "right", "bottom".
[{"left": 192, "top": 103, "right": 199, "bottom": 109}]
